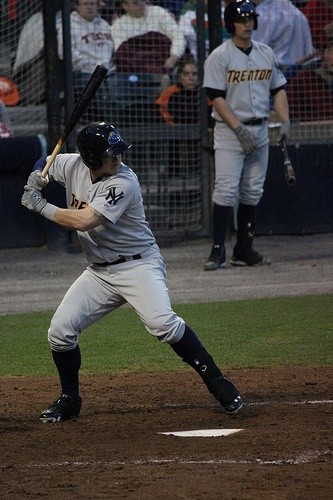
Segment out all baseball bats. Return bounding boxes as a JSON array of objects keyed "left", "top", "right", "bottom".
[
  {"left": 42, "top": 64, "right": 109, "bottom": 179},
  {"left": 281, "top": 139, "right": 297, "bottom": 186}
]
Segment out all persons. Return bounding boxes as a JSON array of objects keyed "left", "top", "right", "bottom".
[
  {"left": 248, "top": 0, "right": 333, "bottom": 120},
  {"left": 20, "top": 121, "right": 244, "bottom": 422},
  {"left": 111, "top": 0, "right": 188, "bottom": 75},
  {"left": 203, "top": 0, "right": 292, "bottom": 270},
  {"left": 155, "top": 58, "right": 216, "bottom": 128},
  {"left": 179, "top": 0, "right": 233, "bottom": 61},
  {"left": 58, "top": 0, "right": 117, "bottom": 108}
]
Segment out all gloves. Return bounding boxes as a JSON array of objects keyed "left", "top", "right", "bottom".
[
  {"left": 21, "top": 170, "right": 50, "bottom": 215},
  {"left": 275, "top": 121, "right": 291, "bottom": 148},
  {"left": 231, "top": 124, "right": 258, "bottom": 155}
]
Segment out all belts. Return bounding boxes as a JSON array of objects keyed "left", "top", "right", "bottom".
[
  {"left": 92, "top": 253, "right": 142, "bottom": 268},
  {"left": 242, "top": 117, "right": 268, "bottom": 126}
]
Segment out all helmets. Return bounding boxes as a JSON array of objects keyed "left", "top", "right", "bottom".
[
  {"left": 224, "top": 0, "right": 259, "bottom": 33},
  {"left": 75, "top": 122, "right": 132, "bottom": 170}
]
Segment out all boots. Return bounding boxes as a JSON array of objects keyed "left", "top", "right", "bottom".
[
  {"left": 203, "top": 202, "right": 233, "bottom": 271},
  {"left": 231, "top": 202, "right": 264, "bottom": 266},
  {"left": 38, "top": 344, "right": 82, "bottom": 423},
  {"left": 170, "top": 324, "right": 243, "bottom": 413}
]
